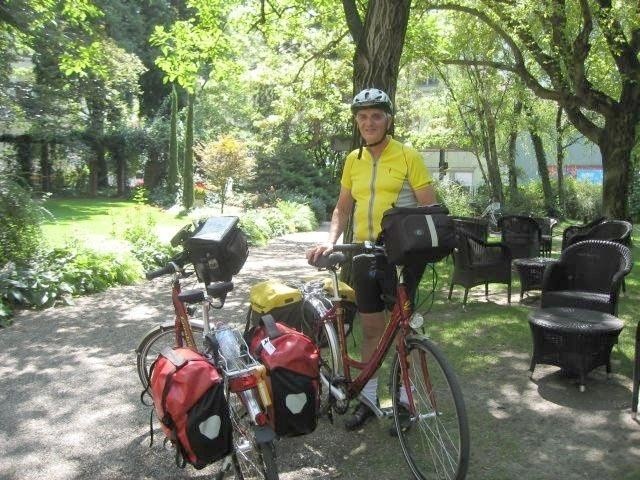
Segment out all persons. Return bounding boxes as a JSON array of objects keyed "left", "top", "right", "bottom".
[{"left": 306, "top": 88, "right": 438, "bottom": 436}]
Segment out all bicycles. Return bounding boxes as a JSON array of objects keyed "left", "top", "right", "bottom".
[
  {"left": 135, "top": 254, "right": 281, "bottom": 479},
  {"left": 286, "top": 240, "right": 469, "bottom": 479}
]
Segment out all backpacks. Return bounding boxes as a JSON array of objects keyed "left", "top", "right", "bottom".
[
  {"left": 248, "top": 280, "right": 301, "bottom": 327},
  {"left": 304, "top": 278, "right": 355, "bottom": 345},
  {"left": 244, "top": 315, "right": 322, "bottom": 439},
  {"left": 142, "top": 347, "right": 232, "bottom": 470}
]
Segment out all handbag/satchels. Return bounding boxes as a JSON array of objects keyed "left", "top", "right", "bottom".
[
  {"left": 381, "top": 204, "right": 457, "bottom": 264},
  {"left": 189, "top": 215, "right": 249, "bottom": 282}
]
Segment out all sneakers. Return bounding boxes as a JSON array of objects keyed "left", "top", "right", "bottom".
[
  {"left": 391, "top": 407, "right": 410, "bottom": 436},
  {"left": 346, "top": 395, "right": 380, "bottom": 430}
]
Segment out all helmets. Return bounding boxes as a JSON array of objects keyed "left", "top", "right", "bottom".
[{"left": 351, "top": 88, "right": 395, "bottom": 115}]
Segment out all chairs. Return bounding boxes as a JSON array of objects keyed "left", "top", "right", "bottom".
[{"left": 452, "top": 210, "right": 632, "bottom": 385}]
